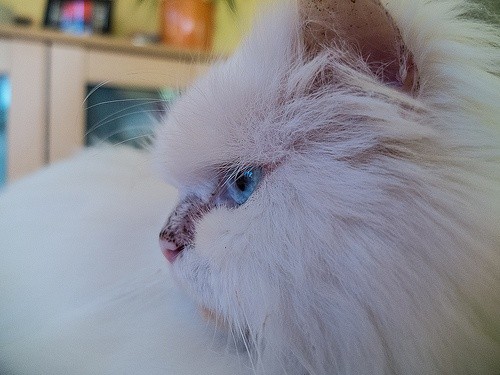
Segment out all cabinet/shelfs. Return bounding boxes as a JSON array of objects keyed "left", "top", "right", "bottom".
[{"left": 0, "top": 24, "right": 231, "bottom": 186}]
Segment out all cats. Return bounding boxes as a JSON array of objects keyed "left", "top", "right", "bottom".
[{"left": 78, "top": 0, "right": 500, "bottom": 375}]
